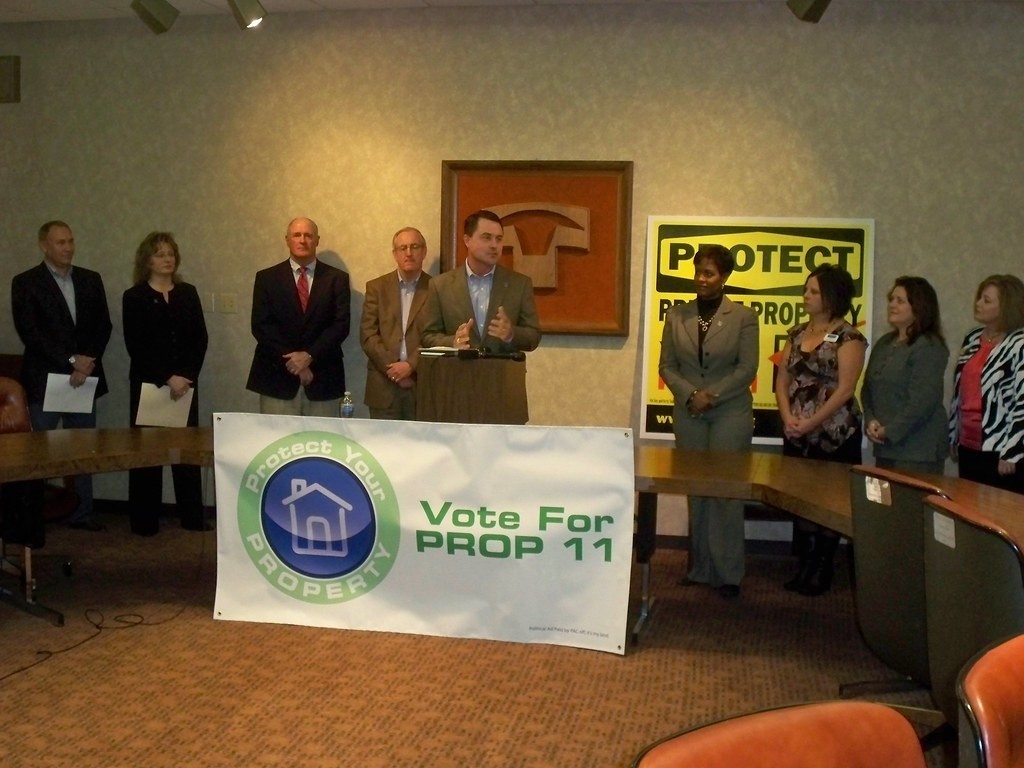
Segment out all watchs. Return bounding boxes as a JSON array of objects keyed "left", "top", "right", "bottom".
[
  {"left": 69, "top": 353, "right": 77, "bottom": 366},
  {"left": 308, "top": 353, "right": 313, "bottom": 361}
]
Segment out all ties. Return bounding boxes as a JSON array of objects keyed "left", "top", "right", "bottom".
[{"left": 296, "top": 268, "right": 309, "bottom": 314}]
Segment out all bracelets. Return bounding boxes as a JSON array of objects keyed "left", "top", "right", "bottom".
[{"left": 688, "top": 390, "right": 698, "bottom": 403}]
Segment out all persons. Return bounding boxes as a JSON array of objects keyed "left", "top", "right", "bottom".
[
  {"left": 775, "top": 262, "right": 869, "bottom": 598},
  {"left": 859, "top": 275, "right": 950, "bottom": 476},
  {"left": 658, "top": 244, "right": 760, "bottom": 595},
  {"left": 9, "top": 220, "right": 114, "bottom": 547},
  {"left": 420, "top": 209, "right": 543, "bottom": 355},
  {"left": 359, "top": 227, "right": 434, "bottom": 419},
  {"left": 949, "top": 273, "right": 1024, "bottom": 496},
  {"left": 122, "top": 231, "right": 214, "bottom": 537},
  {"left": 244, "top": 218, "right": 352, "bottom": 417}
]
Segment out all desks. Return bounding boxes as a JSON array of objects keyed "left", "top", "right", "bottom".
[{"left": 0, "top": 427, "right": 1024, "bottom": 629}]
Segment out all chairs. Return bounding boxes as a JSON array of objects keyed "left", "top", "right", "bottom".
[
  {"left": 0, "top": 352, "right": 45, "bottom": 592},
  {"left": 956, "top": 634, "right": 1024, "bottom": 768},
  {"left": 630, "top": 695, "right": 925, "bottom": 768},
  {"left": 919, "top": 494, "right": 1024, "bottom": 749},
  {"left": 836, "top": 465, "right": 950, "bottom": 699}
]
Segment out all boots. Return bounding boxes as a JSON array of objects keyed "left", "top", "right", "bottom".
[
  {"left": 783, "top": 530, "right": 822, "bottom": 591},
  {"left": 798, "top": 532, "right": 842, "bottom": 596}
]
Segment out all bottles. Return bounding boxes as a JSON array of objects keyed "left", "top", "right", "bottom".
[{"left": 340, "top": 392, "right": 354, "bottom": 418}]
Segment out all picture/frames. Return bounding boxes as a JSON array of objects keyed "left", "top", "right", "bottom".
[{"left": 438, "top": 159, "right": 633, "bottom": 338}]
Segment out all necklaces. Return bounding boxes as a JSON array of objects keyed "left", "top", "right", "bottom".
[
  {"left": 698, "top": 309, "right": 717, "bottom": 332},
  {"left": 808, "top": 318, "right": 837, "bottom": 334},
  {"left": 986, "top": 330, "right": 1000, "bottom": 343}
]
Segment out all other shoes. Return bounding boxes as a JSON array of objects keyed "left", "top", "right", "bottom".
[
  {"left": 720, "top": 584, "right": 739, "bottom": 598},
  {"left": 68, "top": 514, "right": 104, "bottom": 531},
  {"left": 134, "top": 524, "right": 161, "bottom": 536},
  {"left": 184, "top": 521, "right": 214, "bottom": 531},
  {"left": 677, "top": 578, "right": 698, "bottom": 585}
]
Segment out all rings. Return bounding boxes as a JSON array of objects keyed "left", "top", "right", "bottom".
[
  {"left": 183, "top": 391, "right": 186, "bottom": 394},
  {"left": 392, "top": 377, "right": 395, "bottom": 379}
]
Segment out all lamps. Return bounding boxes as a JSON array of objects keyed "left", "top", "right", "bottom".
[
  {"left": 226, "top": 0, "right": 268, "bottom": 30},
  {"left": 783, "top": 0, "right": 832, "bottom": 24},
  {"left": 129, "top": 0, "right": 180, "bottom": 33}
]
